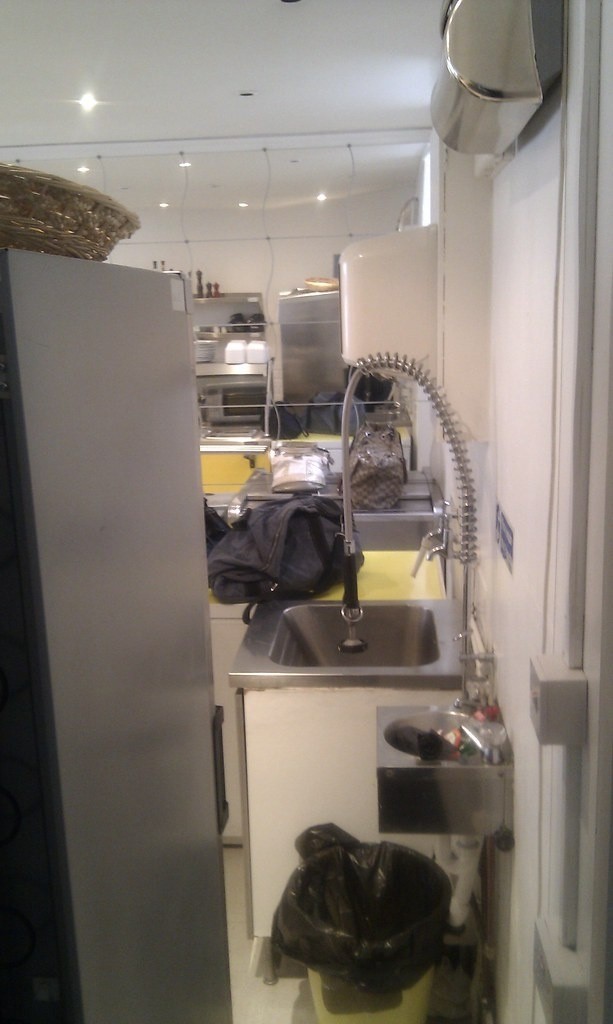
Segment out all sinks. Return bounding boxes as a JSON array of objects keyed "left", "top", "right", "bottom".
[
  {"left": 373, "top": 704, "right": 516, "bottom": 839},
  {"left": 223, "top": 599, "right": 465, "bottom": 691}
]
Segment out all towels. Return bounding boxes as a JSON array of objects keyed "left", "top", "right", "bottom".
[{"left": 205, "top": 495, "right": 365, "bottom": 606}]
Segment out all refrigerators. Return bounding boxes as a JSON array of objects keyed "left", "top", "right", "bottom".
[
  {"left": 278, "top": 290, "right": 364, "bottom": 435},
  {"left": 0, "top": 244, "right": 232, "bottom": 1023}
]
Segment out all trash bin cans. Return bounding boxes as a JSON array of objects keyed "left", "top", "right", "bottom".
[{"left": 283, "top": 840, "right": 452, "bottom": 1024}]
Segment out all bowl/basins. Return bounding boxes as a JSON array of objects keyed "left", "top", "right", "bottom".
[{"left": 224, "top": 339, "right": 268, "bottom": 364}]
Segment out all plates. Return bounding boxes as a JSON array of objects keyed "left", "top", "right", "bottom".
[{"left": 194, "top": 340, "right": 218, "bottom": 363}]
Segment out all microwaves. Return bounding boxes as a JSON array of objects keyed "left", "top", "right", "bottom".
[{"left": 205, "top": 381, "right": 267, "bottom": 425}]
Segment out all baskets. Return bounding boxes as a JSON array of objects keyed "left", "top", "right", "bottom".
[{"left": 0, "top": 160, "right": 142, "bottom": 262}]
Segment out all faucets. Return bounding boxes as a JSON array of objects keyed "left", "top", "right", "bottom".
[
  {"left": 427, "top": 528, "right": 463, "bottom": 564},
  {"left": 451, "top": 697, "right": 490, "bottom": 719},
  {"left": 420, "top": 500, "right": 462, "bottom": 544},
  {"left": 339, "top": 353, "right": 479, "bottom": 707}
]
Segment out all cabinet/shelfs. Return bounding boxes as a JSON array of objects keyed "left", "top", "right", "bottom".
[
  {"left": 231, "top": 687, "right": 466, "bottom": 986},
  {"left": 191, "top": 292, "right": 274, "bottom": 442}
]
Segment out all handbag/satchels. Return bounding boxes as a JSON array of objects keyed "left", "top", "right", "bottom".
[
  {"left": 208, "top": 491, "right": 364, "bottom": 625},
  {"left": 304, "top": 390, "right": 365, "bottom": 436},
  {"left": 343, "top": 365, "right": 377, "bottom": 413},
  {"left": 258, "top": 400, "right": 310, "bottom": 441},
  {"left": 337, "top": 418, "right": 408, "bottom": 511},
  {"left": 246, "top": 312, "right": 264, "bottom": 332},
  {"left": 228, "top": 313, "right": 246, "bottom": 332},
  {"left": 267, "top": 441, "right": 334, "bottom": 492},
  {"left": 372, "top": 370, "right": 400, "bottom": 409},
  {"left": 202, "top": 497, "right": 232, "bottom": 555}
]
